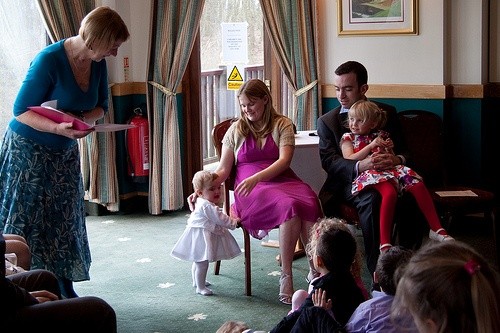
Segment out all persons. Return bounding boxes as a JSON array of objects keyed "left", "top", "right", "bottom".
[
  {"left": 1, "top": 234, "right": 32, "bottom": 277},
  {"left": 187, "top": 78, "right": 321, "bottom": 304},
  {"left": 0, "top": 5, "right": 130, "bottom": 300},
  {"left": 288, "top": 238, "right": 370, "bottom": 316},
  {"left": 309, "top": 246, "right": 420, "bottom": 333},
  {"left": 0, "top": 233, "right": 117, "bottom": 333},
  {"left": 339, "top": 99, "right": 455, "bottom": 253},
  {"left": 316, "top": 61, "right": 439, "bottom": 298},
  {"left": 169, "top": 169, "right": 242, "bottom": 295},
  {"left": 305, "top": 226, "right": 366, "bottom": 327},
  {"left": 389, "top": 239, "right": 500, "bottom": 333}
]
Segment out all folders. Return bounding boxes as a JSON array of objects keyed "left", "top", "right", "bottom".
[{"left": 26, "top": 100, "right": 135, "bottom": 135}]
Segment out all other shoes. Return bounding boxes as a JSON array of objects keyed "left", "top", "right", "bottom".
[
  {"left": 378, "top": 243, "right": 393, "bottom": 254},
  {"left": 429, "top": 228, "right": 455, "bottom": 242}
]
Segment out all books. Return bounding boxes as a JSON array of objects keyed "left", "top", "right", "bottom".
[{"left": 27, "top": 105, "right": 138, "bottom": 133}]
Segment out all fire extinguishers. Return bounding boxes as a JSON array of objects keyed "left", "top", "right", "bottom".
[{"left": 126, "top": 108, "right": 150, "bottom": 176}]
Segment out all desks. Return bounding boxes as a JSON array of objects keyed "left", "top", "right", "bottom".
[{"left": 260, "top": 130, "right": 328, "bottom": 262}]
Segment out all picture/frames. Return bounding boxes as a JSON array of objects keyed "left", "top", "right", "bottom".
[{"left": 336, "top": 0, "right": 419, "bottom": 37}]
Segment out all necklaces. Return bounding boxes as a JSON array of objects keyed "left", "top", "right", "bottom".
[{"left": 69, "top": 37, "right": 92, "bottom": 74}]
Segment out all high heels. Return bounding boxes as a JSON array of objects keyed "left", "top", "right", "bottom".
[
  {"left": 278, "top": 272, "right": 294, "bottom": 304},
  {"left": 304, "top": 268, "right": 319, "bottom": 283}
]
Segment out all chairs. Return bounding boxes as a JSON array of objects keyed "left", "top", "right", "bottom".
[
  {"left": 211, "top": 117, "right": 281, "bottom": 297},
  {"left": 341, "top": 109, "right": 500, "bottom": 267}
]
